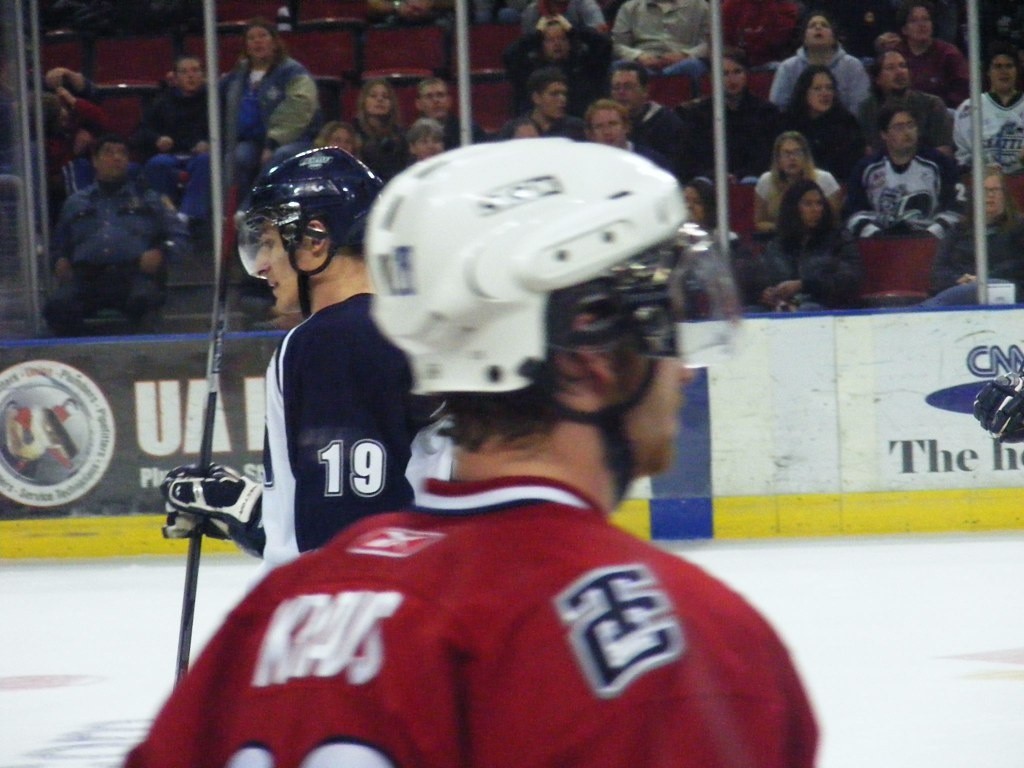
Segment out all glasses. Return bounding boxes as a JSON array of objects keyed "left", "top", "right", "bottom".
[
  {"left": 983, "top": 186, "right": 1004, "bottom": 197},
  {"left": 777, "top": 148, "right": 805, "bottom": 160}
]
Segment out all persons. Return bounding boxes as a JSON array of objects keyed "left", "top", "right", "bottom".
[
  {"left": 156, "top": 146, "right": 427, "bottom": 575},
  {"left": 128, "top": 135, "right": 819, "bottom": 768},
  {"left": 0, "top": 1, "right": 1024, "bottom": 332}
]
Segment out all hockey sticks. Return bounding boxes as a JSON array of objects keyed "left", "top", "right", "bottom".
[{"left": 172, "top": 77, "right": 245, "bottom": 690}]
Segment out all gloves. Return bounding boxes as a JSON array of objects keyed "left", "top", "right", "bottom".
[
  {"left": 972, "top": 371, "right": 1024, "bottom": 443},
  {"left": 161, "top": 460, "right": 264, "bottom": 544}
]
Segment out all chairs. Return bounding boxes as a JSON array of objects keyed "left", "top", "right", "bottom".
[{"left": 45, "top": 0, "right": 936, "bottom": 304}]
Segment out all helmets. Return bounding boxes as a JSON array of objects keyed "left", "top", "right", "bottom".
[
  {"left": 237, "top": 145, "right": 384, "bottom": 281},
  {"left": 363, "top": 137, "right": 740, "bottom": 396}
]
